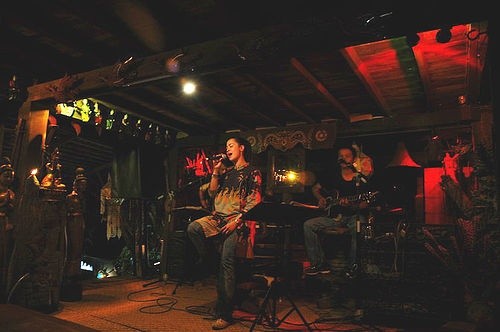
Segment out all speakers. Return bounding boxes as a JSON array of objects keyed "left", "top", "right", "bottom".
[
  {"left": 236, "top": 259, "right": 301, "bottom": 300},
  {"left": 352, "top": 277, "right": 452, "bottom": 329}
]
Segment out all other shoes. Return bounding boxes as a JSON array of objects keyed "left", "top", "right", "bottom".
[
  {"left": 212, "top": 318, "right": 231, "bottom": 330},
  {"left": 304, "top": 265, "right": 332, "bottom": 276}
]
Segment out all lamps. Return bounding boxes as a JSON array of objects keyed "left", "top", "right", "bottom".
[{"left": 80, "top": 99, "right": 173, "bottom": 148}]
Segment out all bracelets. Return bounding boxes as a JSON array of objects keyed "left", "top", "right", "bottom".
[
  {"left": 234, "top": 219, "right": 241, "bottom": 225},
  {"left": 211, "top": 174, "right": 219, "bottom": 178}
]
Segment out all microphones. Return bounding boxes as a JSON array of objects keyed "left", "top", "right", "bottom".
[{"left": 205, "top": 154, "right": 226, "bottom": 161}]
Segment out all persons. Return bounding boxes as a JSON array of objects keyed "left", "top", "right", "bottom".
[
  {"left": 187, "top": 137, "right": 265, "bottom": 330},
  {"left": 0, "top": 156, "right": 16, "bottom": 296},
  {"left": 303, "top": 146, "right": 377, "bottom": 291},
  {"left": 66, "top": 168, "right": 89, "bottom": 274},
  {"left": 456, "top": 138, "right": 480, "bottom": 202}
]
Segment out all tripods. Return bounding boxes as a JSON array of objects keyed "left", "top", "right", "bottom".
[{"left": 239, "top": 201, "right": 319, "bottom": 332}]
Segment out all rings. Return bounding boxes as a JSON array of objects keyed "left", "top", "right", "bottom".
[{"left": 227, "top": 231, "right": 229, "bottom": 233}]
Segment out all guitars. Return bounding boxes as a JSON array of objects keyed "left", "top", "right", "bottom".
[{"left": 319, "top": 189, "right": 379, "bottom": 218}]
